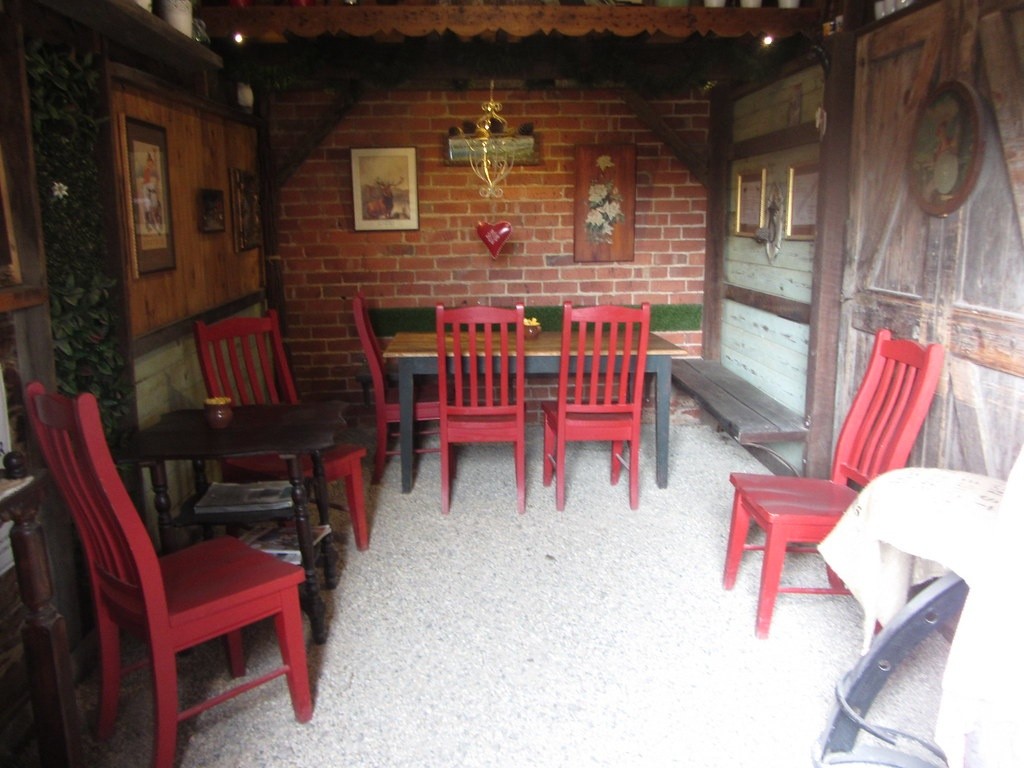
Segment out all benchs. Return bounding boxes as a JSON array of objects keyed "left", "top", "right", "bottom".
[{"left": 669, "top": 358, "right": 806, "bottom": 472}]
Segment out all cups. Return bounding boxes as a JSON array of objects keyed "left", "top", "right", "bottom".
[
  {"left": 204, "top": 396, "right": 232, "bottom": 431},
  {"left": 523, "top": 318, "right": 541, "bottom": 340}
]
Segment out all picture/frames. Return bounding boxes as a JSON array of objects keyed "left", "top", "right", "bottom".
[
  {"left": 197, "top": 189, "right": 225, "bottom": 234},
  {"left": 351, "top": 148, "right": 420, "bottom": 231},
  {"left": 118, "top": 112, "right": 176, "bottom": 278},
  {"left": 229, "top": 167, "right": 265, "bottom": 253}
]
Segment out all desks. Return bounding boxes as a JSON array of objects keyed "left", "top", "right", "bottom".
[
  {"left": 811, "top": 468, "right": 1010, "bottom": 768},
  {"left": 118, "top": 399, "right": 350, "bottom": 646},
  {"left": 382, "top": 333, "right": 686, "bottom": 493}
]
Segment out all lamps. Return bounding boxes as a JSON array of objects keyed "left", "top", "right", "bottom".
[{"left": 457, "top": 78, "right": 522, "bottom": 193}]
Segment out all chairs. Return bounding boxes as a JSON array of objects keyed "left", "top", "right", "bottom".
[
  {"left": 542, "top": 301, "right": 652, "bottom": 509},
  {"left": 435, "top": 302, "right": 527, "bottom": 516},
  {"left": 26, "top": 382, "right": 312, "bottom": 768},
  {"left": 724, "top": 327, "right": 945, "bottom": 641},
  {"left": 194, "top": 310, "right": 369, "bottom": 550},
  {"left": 353, "top": 289, "right": 441, "bottom": 486}
]
